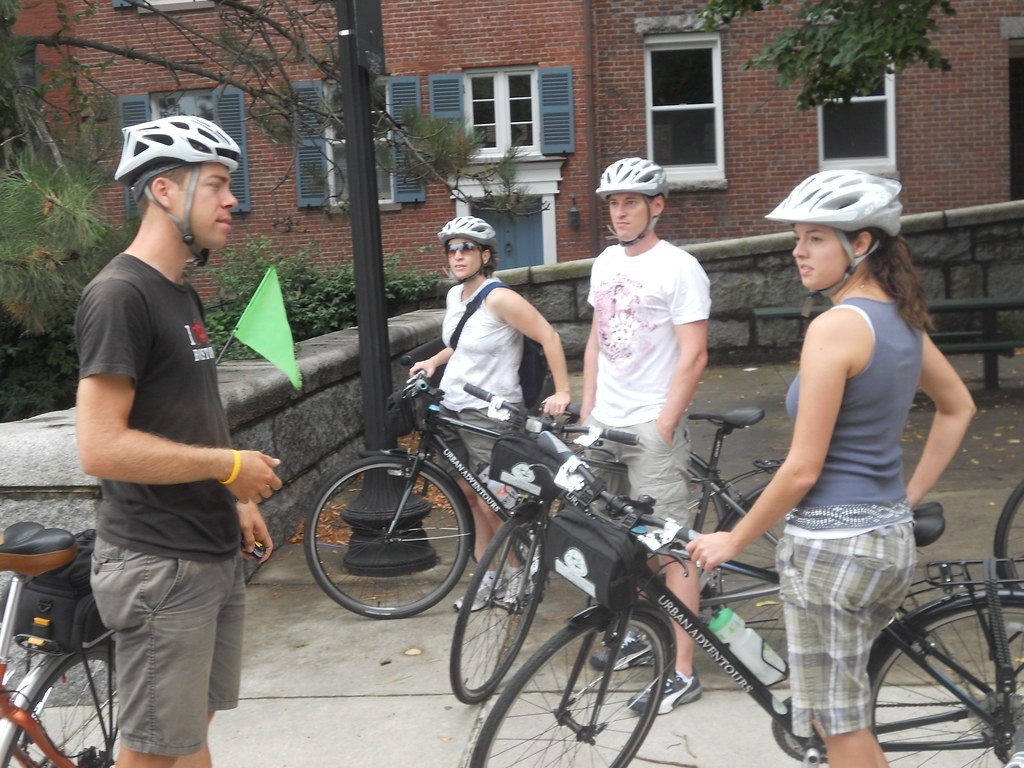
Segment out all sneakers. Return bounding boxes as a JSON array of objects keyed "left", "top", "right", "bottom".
[
  {"left": 628, "top": 666, "right": 701, "bottom": 714},
  {"left": 454, "top": 577, "right": 505, "bottom": 611},
  {"left": 591, "top": 630, "right": 658, "bottom": 670},
  {"left": 504, "top": 562, "right": 534, "bottom": 603}
]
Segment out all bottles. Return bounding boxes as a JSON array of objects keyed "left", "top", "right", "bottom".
[
  {"left": 476, "top": 462, "right": 519, "bottom": 510},
  {"left": 708, "top": 606, "right": 787, "bottom": 686}
]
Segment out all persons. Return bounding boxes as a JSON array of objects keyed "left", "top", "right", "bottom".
[
  {"left": 580, "top": 156, "right": 712, "bottom": 715},
  {"left": 685, "top": 168, "right": 977, "bottom": 768},
  {"left": 75, "top": 115, "right": 281, "bottom": 768},
  {"left": 409, "top": 215, "right": 571, "bottom": 612}
]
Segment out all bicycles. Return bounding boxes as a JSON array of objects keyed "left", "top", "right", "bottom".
[
  {"left": 0, "top": 521, "right": 123, "bottom": 768},
  {"left": 459, "top": 418, "right": 1023, "bottom": 766},
  {"left": 449, "top": 382, "right": 788, "bottom": 704},
  {"left": 303, "top": 371, "right": 579, "bottom": 619}
]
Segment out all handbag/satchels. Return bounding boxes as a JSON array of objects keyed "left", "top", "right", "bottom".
[
  {"left": 14, "top": 529, "right": 116, "bottom": 650},
  {"left": 519, "top": 335, "right": 547, "bottom": 408}
]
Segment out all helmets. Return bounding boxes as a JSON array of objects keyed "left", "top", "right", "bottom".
[
  {"left": 113, "top": 114, "right": 241, "bottom": 185},
  {"left": 596, "top": 157, "right": 668, "bottom": 201},
  {"left": 764, "top": 169, "right": 903, "bottom": 237},
  {"left": 438, "top": 216, "right": 496, "bottom": 248}
]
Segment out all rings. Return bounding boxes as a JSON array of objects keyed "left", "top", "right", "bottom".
[
  {"left": 695, "top": 559, "right": 705, "bottom": 568},
  {"left": 555, "top": 410, "right": 560, "bottom": 414}
]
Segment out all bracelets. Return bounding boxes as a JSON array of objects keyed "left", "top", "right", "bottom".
[{"left": 218, "top": 448, "right": 240, "bottom": 485}]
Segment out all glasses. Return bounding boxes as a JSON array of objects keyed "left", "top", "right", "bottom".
[{"left": 447, "top": 242, "right": 484, "bottom": 257}]
